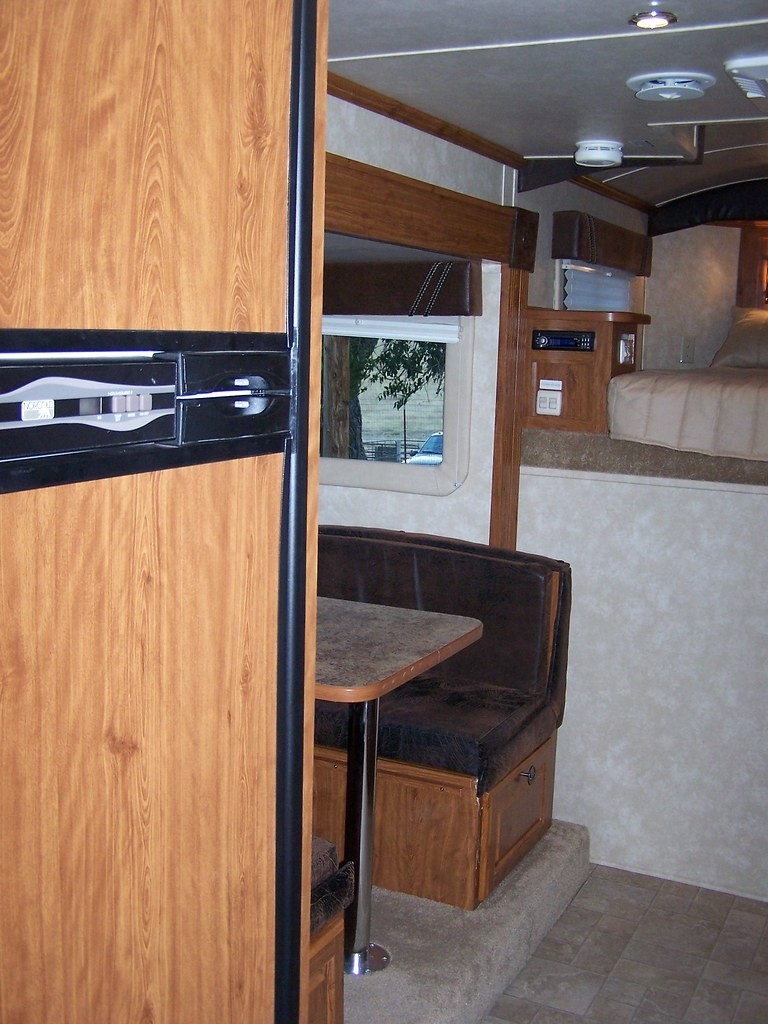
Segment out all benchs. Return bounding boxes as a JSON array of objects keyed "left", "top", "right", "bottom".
[{"left": 312, "top": 523, "right": 573, "bottom": 907}]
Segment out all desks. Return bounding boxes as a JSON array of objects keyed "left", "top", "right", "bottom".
[{"left": 315, "top": 596, "right": 485, "bottom": 977}]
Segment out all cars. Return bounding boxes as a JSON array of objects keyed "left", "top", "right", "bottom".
[{"left": 401, "top": 432, "right": 444, "bottom": 465}]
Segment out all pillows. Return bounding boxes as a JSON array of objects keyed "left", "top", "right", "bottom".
[{"left": 708, "top": 306, "right": 768, "bottom": 368}]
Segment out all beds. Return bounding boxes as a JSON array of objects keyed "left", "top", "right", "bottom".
[{"left": 608, "top": 367, "right": 768, "bottom": 461}]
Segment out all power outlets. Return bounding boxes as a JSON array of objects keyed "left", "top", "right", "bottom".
[{"left": 681, "top": 335, "right": 696, "bottom": 363}]
